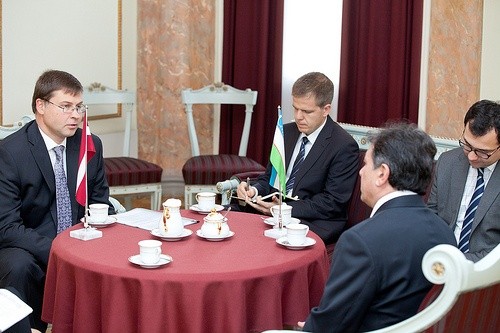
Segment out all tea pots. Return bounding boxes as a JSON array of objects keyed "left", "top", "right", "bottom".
[
  {"left": 201, "top": 208, "right": 230, "bottom": 236},
  {"left": 158, "top": 197, "right": 183, "bottom": 236}
]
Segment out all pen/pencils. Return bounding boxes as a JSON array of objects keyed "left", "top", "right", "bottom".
[{"left": 246, "top": 177, "right": 250, "bottom": 193}]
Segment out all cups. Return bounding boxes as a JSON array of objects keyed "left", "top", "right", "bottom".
[
  {"left": 86, "top": 204, "right": 109, "bottom": 222},
  {"left": 137, "top": 240, "right": 162, "bottom": 264},
  {"left": 286, "top": 224, "right": 309, "bottom": 245},
  {"left": 195, "top": 192, "right": 216, "bottom": 209},
  {"left": 271, "top": 204, "right": 292, "bottom": 225}
]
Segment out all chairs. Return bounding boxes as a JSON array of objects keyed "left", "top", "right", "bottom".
[
  {"left": 82, "top": 83, "right": 164, "bottom": 210},
  {"left": 182, "top": 82, "right": 265, "bottom": 210},
  {"left": 362, "top": 242, "right": 500, "bottom": 333}
]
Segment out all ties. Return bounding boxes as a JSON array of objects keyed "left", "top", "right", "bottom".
[
  {"left": 52, "top": 145, "right": 72, "bottom": 234},
  {"left": 459, "top": 167, "right": 485, "bottom": 254},
  {"left": 282, "top": 136, "right": 308, "bottom": 195}
]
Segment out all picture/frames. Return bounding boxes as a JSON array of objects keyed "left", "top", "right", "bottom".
[{"left": 0, "top": 0, "right": 122, "bottom": 128}]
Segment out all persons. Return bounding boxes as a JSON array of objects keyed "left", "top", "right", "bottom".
[
  {"left": 428, "top": 99, "right": 500, "bottom": 265},
  {"left": 220, "top": 72, "right": 360, "bottom": 244},
  {"left": 0, "top": 285, "right": 32, "bottom": 333},
  {"left": 297, "top": 124, "right": 457, "bottom": 333},
  {"left": 0, "top": 70, "right": 116, "bottom": 333}
]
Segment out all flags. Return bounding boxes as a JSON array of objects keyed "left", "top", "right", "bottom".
[
  {"left": 269, "top": 112, "right": 286, "bottom": 199},
  {"left": 75, "top": 111, "right": 96, "bottom": 207}
]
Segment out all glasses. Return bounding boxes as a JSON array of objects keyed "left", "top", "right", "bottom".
[
  {"left": 459, "top": 129, "right": 500, "bottom": 159},
  {"left": 41, "top": 96, "right": 88, "bottom": 113}
]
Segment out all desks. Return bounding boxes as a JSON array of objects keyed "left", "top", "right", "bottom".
[{"left": 41, "top": 206, "right": 336, "bottom": 333}]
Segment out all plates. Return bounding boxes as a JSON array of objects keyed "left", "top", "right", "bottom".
[
  {"left": 195, "top": 229, "right": 236, "bottom": 242},
  {"left": 80, "top": 216, "right": 118, "bottom": 227},
  {"left": 151, "top": 227, "right": 194, "bottom": 242},
  {"left": 264, "top": 217, "right": 301, "bottom": 227},
  {"left": 128, "top": 254, "right": 173, "bottom": 268},
  {"left": 276, "top": 236, "right": 316, "bottom": 250},
  {"left": 190, "top": 203, "right": 224, "bottom": 215}
]
